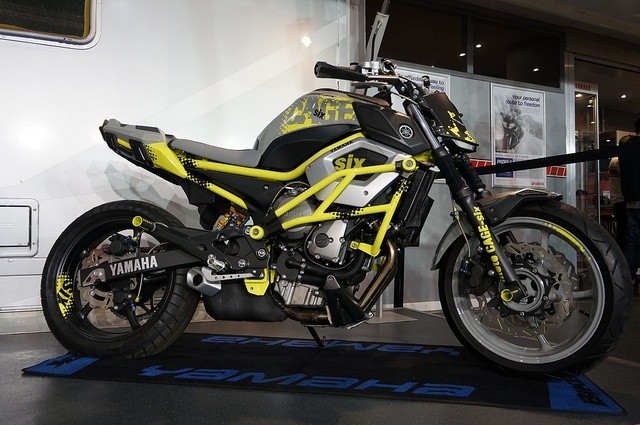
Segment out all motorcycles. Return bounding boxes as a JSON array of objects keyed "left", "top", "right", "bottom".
[
  {"left": 36, "top": 53, "right": 635, "bottom": 387},
  {"left": 500, "top": 108, "right": 524, "bottom": 151}
]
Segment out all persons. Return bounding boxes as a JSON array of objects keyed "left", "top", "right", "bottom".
[
  {"left": 421, "top": 74, "right": 434, "bottom": 90},
  {"left": 608, "top": 134, "right": 633, "bottom": 259},
  {"left": 618, "top": 118, "right": 640, "bottom": 301}
]
